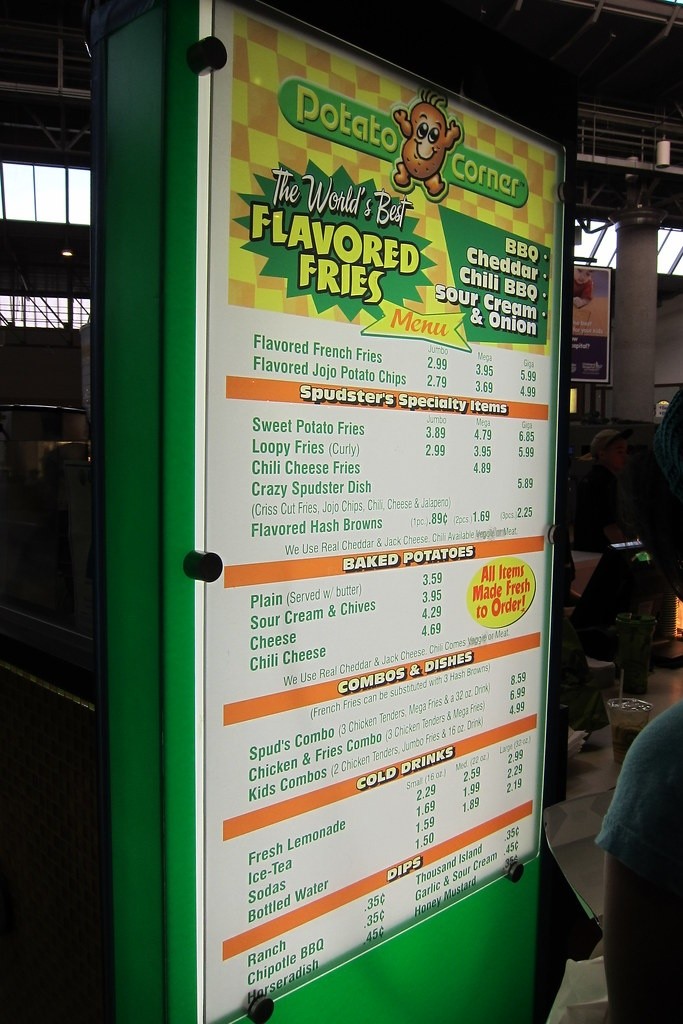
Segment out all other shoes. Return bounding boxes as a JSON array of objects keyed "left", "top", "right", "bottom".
[{"left": 585, "top": 655, "right": 615, "bottom": 674}]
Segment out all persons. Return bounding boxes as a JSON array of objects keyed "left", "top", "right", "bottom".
[{"left": 548, "top": 380, "right": 683, "bottom": 1024}]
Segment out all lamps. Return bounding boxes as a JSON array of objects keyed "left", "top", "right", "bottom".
[
  {"left": 656, "top": 134, "right": 670, "bottom": 169},
  {"left": 624, "top": 156, "right": 640, "bottom": 184},
  {"left": 61, "top": 222, "right": 77, "bottom": 257}
]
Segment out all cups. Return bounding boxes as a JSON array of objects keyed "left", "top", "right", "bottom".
[
  {"left": 615, "top": 613, "right": 657, "bottom": 695},
  {"left": 607, "top": 698, "right": 654, "bottom": 764}
]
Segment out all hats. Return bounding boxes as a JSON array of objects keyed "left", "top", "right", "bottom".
[
  {"left": 652, "top": 390, "right": 683, "bottom": 502},
  {"left": 591, "top": 429, "right": 633, "bottom": 456}
]
